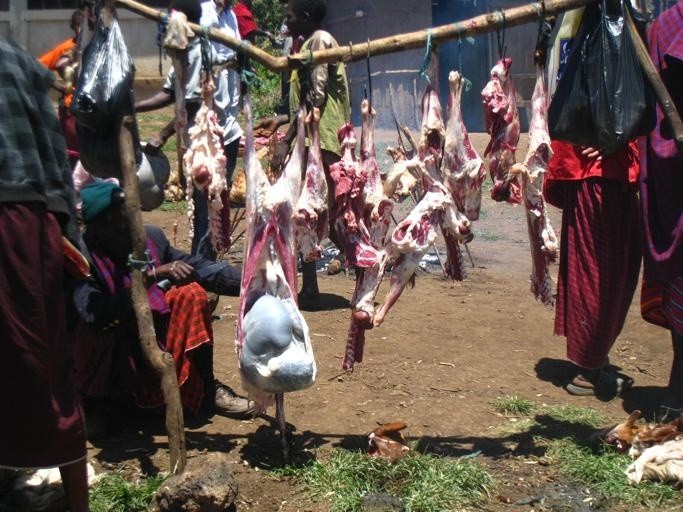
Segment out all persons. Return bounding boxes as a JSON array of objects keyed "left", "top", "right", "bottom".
[
  {"left": 57, "top": 203, "right": 261, "bottom": 426},
  {"left": 51, "top": 6, "right": 142, "bottom": 180},
  {"left": 637, "top": 2, "right": 683, "bottom": 412},
  {"left": 0, "top": 28, "right": 93, "bottom": 512},
  {"left": 135, "top": 1, "right": 258, "bottom": 261},
  {"left": 73, "top": 160, "right": 242, "bottom": 296},
  {"left": 253, "top": 1, "right": 350, "bottom": 307},
  {"left": 540, "top": 118, "right": 652, "bottom": 399}
]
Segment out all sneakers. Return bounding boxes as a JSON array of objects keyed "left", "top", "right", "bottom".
[
  {"left": 214, "top": 380, "right": 264, "bottom": 417},
  {"left": 564, "top": 371, "right": 632, "bottom": 397}
]
